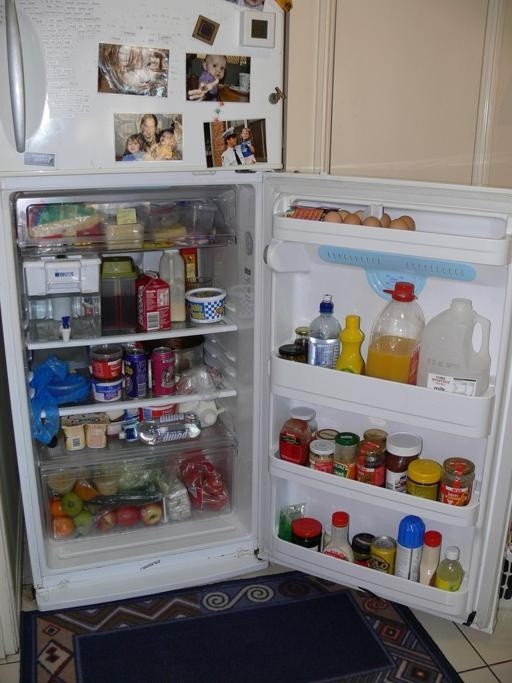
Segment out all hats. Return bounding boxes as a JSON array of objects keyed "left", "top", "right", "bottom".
[{"left": 222, "top": 127, "right": 234, "bottom": 138}]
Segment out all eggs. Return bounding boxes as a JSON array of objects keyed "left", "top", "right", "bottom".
[{"left": 324, "top": 210, "right": 415, "bottom": 231}]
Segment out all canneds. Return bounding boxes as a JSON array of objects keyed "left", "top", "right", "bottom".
[
  {"left": 309, "top": 428, "right": 475, "bottom": 507},
  {"left": 292, "top": 518, "right": 322, "bottom": 552},
  {"left": 90, "top": 344, "right": 177, "bottom": 401},
  {"left": 351, "top": 533, "right": 396, "bottom": 575},
  {"left": 278, "top": 327, "right": 309, "bottom": 363}
]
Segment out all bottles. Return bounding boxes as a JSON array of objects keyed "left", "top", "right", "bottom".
[
  {"left": 336, "top": 315, "right": 364, "bottom": 373},
  {"left": 417, "top": 530, "right": 445, "bottom": 584},
  {"left": 334, "top": 427, "right": 359, "bottom": 479},
  {"left": 434, "top": 546, "right": 464, "bottom": 593},
  {"left": 306, "top": 294, "right": 340, "bottom": 368},
  {"left": 364, "top": 281, "right": 427, "bottom": 386},
  {"left": 294, "top": 326, "right": 309, "bottom": 363},
  {"left": 321, "top": 512, "right": 353, "bottom": 561}
]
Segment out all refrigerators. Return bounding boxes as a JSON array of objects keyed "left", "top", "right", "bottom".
[{"left": 0, "top": 2, "right": 512, "bottom": 635}]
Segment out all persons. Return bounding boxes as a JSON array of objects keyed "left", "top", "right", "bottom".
[
  {"left": 123, "top": 114, "right": 162, "bottom": 156},
  {"left": 121, "top": 134, "right": 148, "bottom": 161},
  {"left": 98, "top": 44, "right": 167, "bottom": 96},
  {"left": 241, "top": 127, "right": 256, "bottom": 165},
  {"left": 220, "top": 126, "right": 243, "bottom": 168},
  {"left": 147, "top": 129, "right": 175, "bottom": 159},
  {"left": 188, "top": 54, "right": 227, "bottom": 102}
]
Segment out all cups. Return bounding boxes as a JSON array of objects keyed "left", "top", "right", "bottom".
[{"left": 185, "top": 274, "right": 213, "bottom": 289}]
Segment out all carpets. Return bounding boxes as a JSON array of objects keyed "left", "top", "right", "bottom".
[{"left": 19, "top": 567, "right": 467, "bottom": 683}]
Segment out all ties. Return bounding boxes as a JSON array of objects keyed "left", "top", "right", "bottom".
[{"left": 233, "top": 148, "right": 242, "bottom": 165}]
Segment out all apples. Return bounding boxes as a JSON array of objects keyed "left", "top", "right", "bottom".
[{"left": 62, "top": 482, "right": 163, "bottom": 532}]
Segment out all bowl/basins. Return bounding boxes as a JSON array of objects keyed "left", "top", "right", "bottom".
[{"left": 44, "top": 373, "right": 94, "bottom": 405}]
